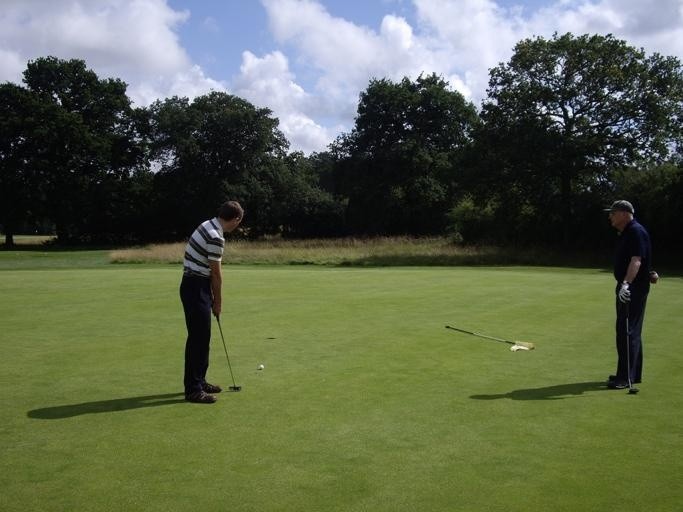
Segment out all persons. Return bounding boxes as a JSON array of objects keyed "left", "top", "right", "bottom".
[
  {"left": 179, "top": 201, "right": 244, "bottom": 403},
  {"left": 603, "top": 200, "right": 659, "bottom": 389}
]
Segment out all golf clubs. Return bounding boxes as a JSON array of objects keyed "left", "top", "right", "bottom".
[
  {"left": 212, "top": 304, "right": 242, "bottom": 390},
  {"left": 624, "top": 301, "right": 639, "bottom": 394}
]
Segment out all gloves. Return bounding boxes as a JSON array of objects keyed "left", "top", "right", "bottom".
[{"left": 619, "top": 280, "right": 631, "bottom": 303}]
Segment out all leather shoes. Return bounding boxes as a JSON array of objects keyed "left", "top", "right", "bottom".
[
  {"left": 608, "top": 375, "right": 631, "bottom": 390},
  {"left": 185, "top": 383, "right": 222, "bottom": 404}
]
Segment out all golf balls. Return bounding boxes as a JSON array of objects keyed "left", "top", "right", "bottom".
[{"left": 259, "top": 364, "right": 264, "bottom": 370}]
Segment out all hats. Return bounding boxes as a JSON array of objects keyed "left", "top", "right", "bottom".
[{"left": 604, "top": 200, "right": 634, "bottom": 214}]
